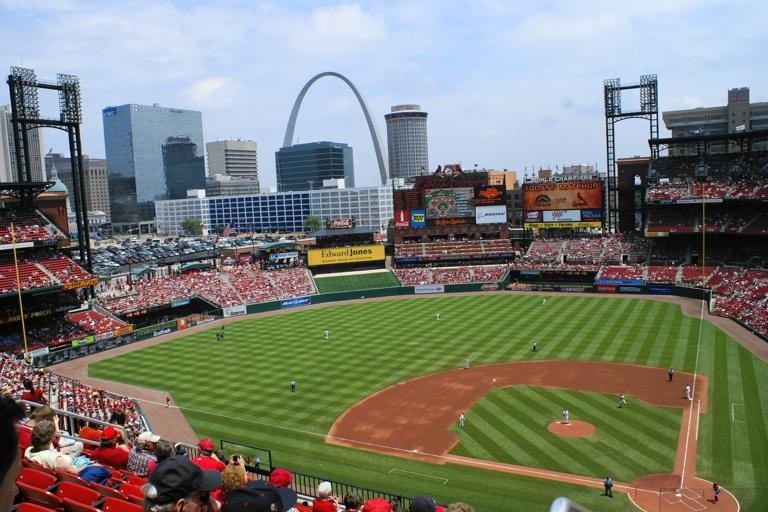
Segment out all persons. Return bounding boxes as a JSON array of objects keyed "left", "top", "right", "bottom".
[
  {"left": 435, "top": 313, "right": 441, "bottom": 320},
  {"left": 458, "top": 412, "right": 465, "bottom": 429},
  {"left": 667, "top": 368, "right": 674, "bottom": 382},
  {"left": 711, "top": 479, "right": 720, "bottom": 503},
  {"left": 154, "top": 431, "right": 476, "bottom": 511},
  {"left": 603, "top": 475, "right": 614, "bottom": 497},
  {"left": 464, "top": 357, "right": 469, "bottom": 368},
  {"left": 165, "top": 391, "right": 171, "bottom": 408},
  {"left": 542, "top": 297, "right": 546, "bottom": 306},
  {"left": 532, "top": 341, "right": 537, "bottom": 352},
  {"left": 0, "top": 203, "right": 315, "bottom": 357},
  {"left": 618, "top": 393, "right": 629, "bottom": 408},
  {"left": 562, "top": 407, "right": 569, "bottom": 423},
  {"left": 290, "top": 379, "right": 297, "bottom": 392},
  {"left": 710, "top": 295, "right": 768, "bottom": 344},
  {"left": 0, "top": 356, "right": 153, "bottom": 511},
  {"left": 393, "top": 151, "right": 768, "bottom": 293},
  {"left": 322, "top": 328, "right": 330, "bottom": 344},
  {"left": 680, "top": 383, "right": 694, "bottom": 400}
]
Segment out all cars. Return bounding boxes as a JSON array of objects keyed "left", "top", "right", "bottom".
[{"left": 72, "top": 232, "right": 296, "bottom": 275}]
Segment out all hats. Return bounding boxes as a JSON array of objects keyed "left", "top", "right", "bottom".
[
  {"left": 101, "top": 426, "right": 120, "bottom": 440},
  {"left": 362, "top": 498, "right": 392, "bottom": 511},
  {"left": 196, "top": 438, "right": 215, "bottom": 450},
  {"left": 143, "top": 453, "right": 223, "bottom": 506},
  {"left": 220, "top": 478, "right": 298, "bottom": 511},
  {"left": 269, "top": 468, "right": 294, "bottom": 488},
  {"left": 137, "top": 431, "right": 161, "bottom": 445},
  {"left": 408, "top": 494, "right": 445, "bottom": 512},
  {"left": 317, "top": 480, "right": 332, "bottom": 497}
]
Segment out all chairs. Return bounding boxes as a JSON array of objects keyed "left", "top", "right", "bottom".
[
  {"left": 92, "top": 265, "right": 319, "bottom": 341},
  {"left": 0, "top": 307, "right": 127, "bottom": 356},
  {"left": 0, "top": 208, "right": 48, "bottom": 243},
  {"left": 394, "top": 179, "right": 767, "bottom": 343},
  {"left": 11, "top": 429, "right": 146, "bottom": 512},
  {"left": 0, "top": 257, "right": 84, "bottom": 296}
]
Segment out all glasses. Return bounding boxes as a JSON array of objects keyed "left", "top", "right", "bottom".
[{"left": 191, "top": 490, "right": 210, "bottom": 505}]
